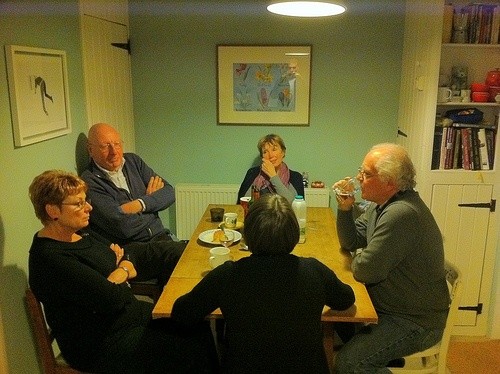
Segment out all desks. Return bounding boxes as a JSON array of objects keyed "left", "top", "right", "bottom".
[{"left": 152, "top": 204, "right": 378, "bottom": 374}]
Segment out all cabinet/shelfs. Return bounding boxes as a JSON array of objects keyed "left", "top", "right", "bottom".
[
  {"left": 175, "top": 185, "right": 329, "bottom": 240},
  {"left": 394, "top": 0, "right": 500, "bottom": 343}
]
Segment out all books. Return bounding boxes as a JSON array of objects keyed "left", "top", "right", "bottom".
[
  {"left": 442, "top": 3, "right": 500, "bottom": 44},
  {"left": 432, "top": 126, "right": 495, "bottom": 171}
]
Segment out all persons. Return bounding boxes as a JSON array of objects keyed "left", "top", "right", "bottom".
[
  {"left": 333, "top": 143, "right": 452, "bottom": 374},
  {"left": 236, "top": 134, "right": 305, "bottom": 206},
  {"left": 80, "top": 123, "right": 189, "bottom": 282},
  {"left": 28, "top": 170, "right": 220, "bottom": 374},
  {"left": 170, "top": 192, "right": 355, "bottom": 374}
]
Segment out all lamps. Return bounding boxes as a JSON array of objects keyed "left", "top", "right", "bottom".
[{"left": 266, "top": 0, "right": 348, "bottom": 18}]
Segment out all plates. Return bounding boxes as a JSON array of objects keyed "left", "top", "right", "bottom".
[
  {"left": 219, "top": 222, "right": 245, "bottom": 229},
  {"left": 198, "top": 229, "right": 242, "bottom": 244}
]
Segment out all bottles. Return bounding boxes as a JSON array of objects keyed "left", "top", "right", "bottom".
[
  {"left": 291, "top": 195, "right": 306, "bottom": 243},
  {"left": 251, "top": 185, "right": 260, "bottom": 199}
]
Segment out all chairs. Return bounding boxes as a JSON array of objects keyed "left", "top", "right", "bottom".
[
  {"left": 23, "top": 276, "right": 168, "bottom": 374},
  {"left": 331, "top": 260, "right": 467, "bottom": 374}
]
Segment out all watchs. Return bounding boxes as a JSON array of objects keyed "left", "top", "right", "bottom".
[{"left": 119, "top": 266, "right": 129, "bottom": 280}]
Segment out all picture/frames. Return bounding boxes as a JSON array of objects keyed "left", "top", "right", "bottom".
[
  {"left": 216, "top": 43, "right": 313, "bottom": 127},
  {"left": 5, "top": 44, "right": 72, "bottom": 148}
]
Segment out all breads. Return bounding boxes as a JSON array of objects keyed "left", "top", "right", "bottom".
[{"left": 212, "top": 230, "right": 226, "bottom": 242}]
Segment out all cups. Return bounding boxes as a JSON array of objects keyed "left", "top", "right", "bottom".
[
  {"left": 334, "top": 176, "right": 362, "bottom": 204},
  {"left": 224, "top": 212, "right": 238, "bottom": 228},
  {"left": 210, "top": 208, "right": 225, "bottom": 222},
  {"left": 240, "top": 196, "right": 252, "bottom": 216},
  {"left": 437, "top": 87, "right": 452, "bottom": 102},
  {"left": 220, "top": 230, "right": 234, "bottom": 247},
  {"left": 209, "top": 247, "right": 231, "bottom": 267},
  {"left": 460, "top": 89, "right": 471, "bottom": 103}
]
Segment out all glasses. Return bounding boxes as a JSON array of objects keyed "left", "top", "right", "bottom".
[
  {"left": 358, "top": 166, "right": 384, "bottom": 179},
  {"left": 53, "top": 199, "right": 91, "bottom": 208},
  {"left": 89, "top": 140, "right": 122, "bottom": 151}
]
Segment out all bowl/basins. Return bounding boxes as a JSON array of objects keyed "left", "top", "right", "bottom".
[{"left": 471, "top": 68, "right": 500, "bottom": 102}]
